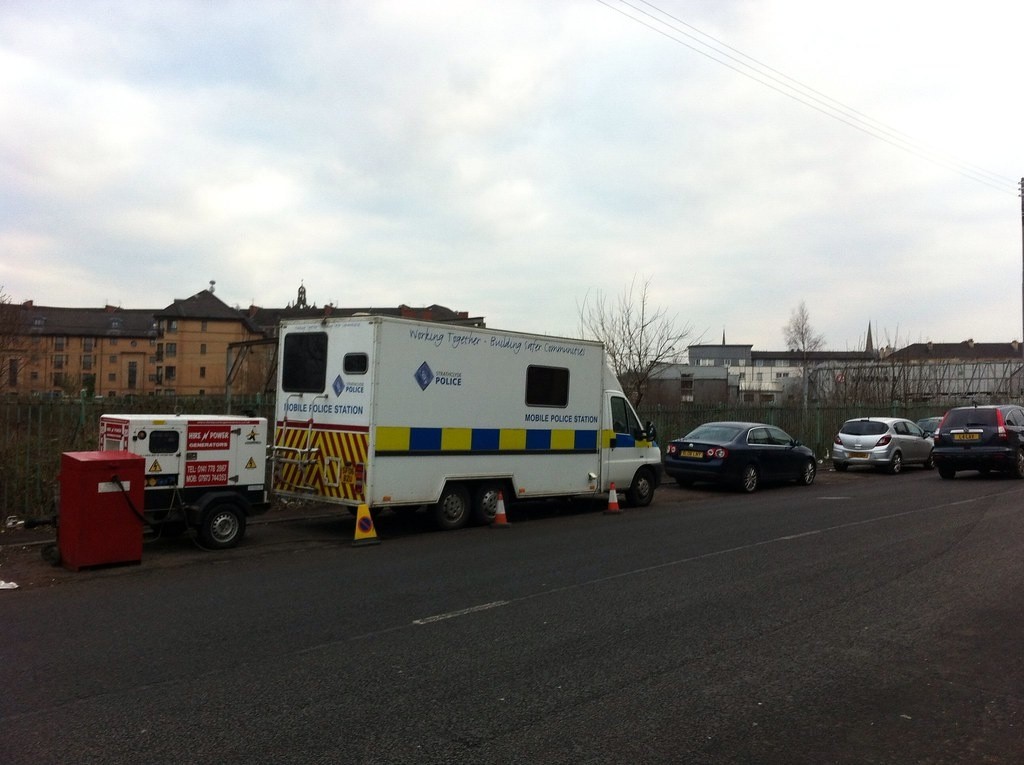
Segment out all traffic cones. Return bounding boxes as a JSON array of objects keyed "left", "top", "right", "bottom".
[
  {"left": 602, "top": 481, "right": 623, "bottom": 515},
  {"left": 350, "top": 503, "right": 382, "bottom": 548},
  {"left": 489, "top": 490, "right": 512, "bottom": 529}
]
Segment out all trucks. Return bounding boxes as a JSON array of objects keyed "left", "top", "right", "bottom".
[{"left": 267, "top": 308, "right": 665, "bottom": 533}]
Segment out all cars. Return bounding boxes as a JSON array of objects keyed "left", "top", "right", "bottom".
[
  {"left": 915, "top": 416, "right": 944, "bottom": 437},
  {"left": 663, "top": 419, "right": 818, "bottom": 494},
  {"left": 830, "top": 416, "right": 937, "bottom": 475}
]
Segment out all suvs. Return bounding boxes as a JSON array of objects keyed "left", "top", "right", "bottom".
[{"left": 930, "top": 405, "right": 1024, "bottom": 481}]
